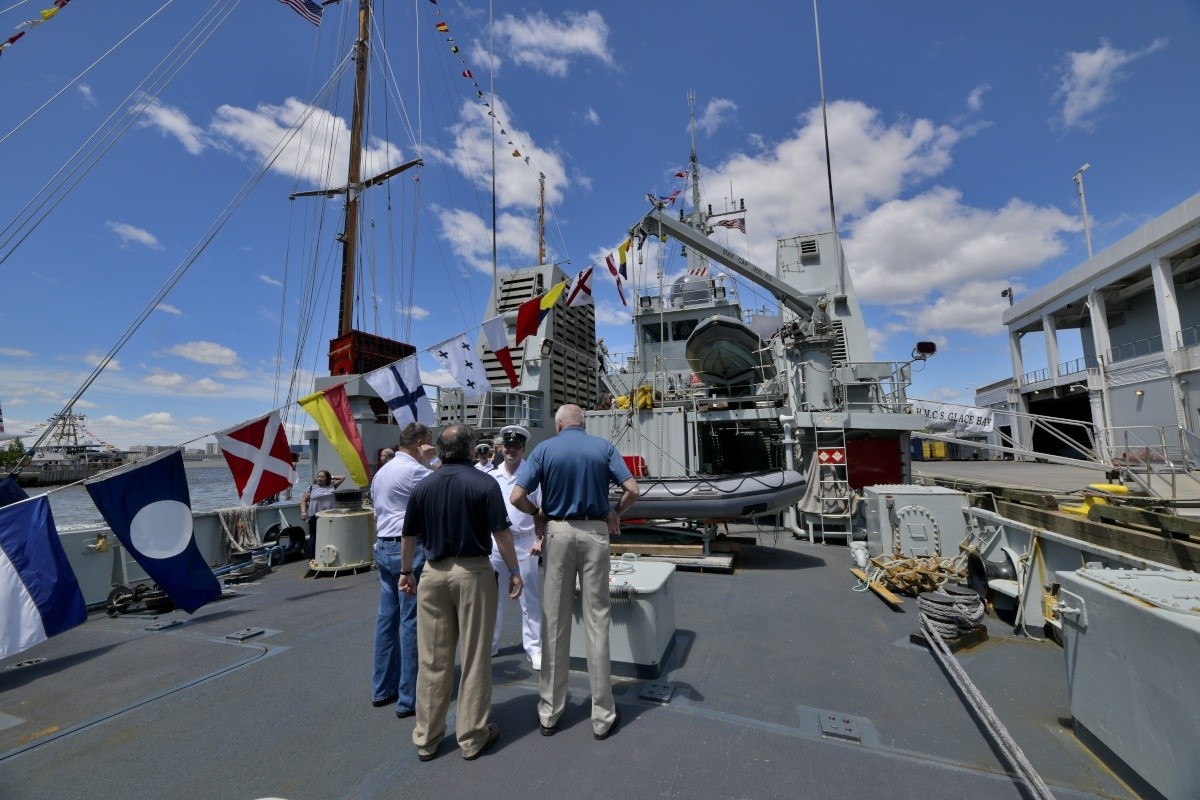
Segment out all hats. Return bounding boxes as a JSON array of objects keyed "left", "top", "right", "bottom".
[
  {"left": 500, "top": 425, "right": 531, "bottom": 443},
  {"left": 475, "top": 444, "right": 490, "bottom": 454}
]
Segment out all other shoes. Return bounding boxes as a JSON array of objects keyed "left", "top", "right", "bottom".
[
  {"left": 418, "top": 742, "right": 441, "bottom": 761},
  {"left": 594, "top": 707, "right": 620, "bottom": 741},
  {"left": 396, "top": 711, "right": 415, "bottom": 718},
  {"left": 372, "top": 694, "right": 399, "bottom": 707},
  {"left": 537, "top": 719, "right": 557, "bottom": 736},
  {"left": 462, "top": 723, "right": 499, "bottom": 760}
]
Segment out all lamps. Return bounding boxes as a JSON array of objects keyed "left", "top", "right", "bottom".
[
  {"left": 713, "top": 287, "right": 726, "bottom": 299},
  {"left": 640, "top": 296, "right": 651, "bottom": 308},
  {"left": 541, "top": 338, "right": 554, "bottom": 355}
]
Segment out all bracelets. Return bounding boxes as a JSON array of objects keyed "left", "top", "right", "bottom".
[
  {"left": 429, "top": 455, "right": 441, "bottom": 467},
  {"left": 399, "top": 568, "right": 413, "bottom": 575},
  {"left": 331, "top": 474, "right": 334, "bottom": 479},
  {"left": 611, "top": 506, "right": 621, "bottom": 517},
  {"left": 530, "top": 506, "right": 540, "bottom": 516},
  {"left": 509, "top": 566, "right": 520, "bottom": 575}
]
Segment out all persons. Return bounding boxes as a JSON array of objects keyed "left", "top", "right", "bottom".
[
  {"left": 510, "top": 404, "right": 640, "bottom": 741},
  {"left": 300, "top": 470, "right": 346, "bottom": 565},
  {"left": 398, "top": 423, "right": 522, "bottom": 761},
  {"left": 371, "top": 422, "right": 442, "bottom": 719},
  {"left": 474, "top": 425, "right": 546, "bottom": 671}
]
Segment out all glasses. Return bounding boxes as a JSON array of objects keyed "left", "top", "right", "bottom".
[{"left": 504, "top": 443, "right": 522, "bottom": 450}]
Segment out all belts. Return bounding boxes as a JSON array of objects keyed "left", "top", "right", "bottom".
[
  {"left": 545, "top": 514, "right": 605, "bottom": 522},
  {"left": 378, "top": 536, "right": 401, "bottom": 542},
  {"left": 446, "top": 553, "right": 488, "bottom": 557}
]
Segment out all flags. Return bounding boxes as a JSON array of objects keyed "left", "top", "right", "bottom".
[
  {"left": 659, "top": 190, "right": 680, "bottom": 205},
  {"left": 1, "top": 496, "right": 87, "bottom": 659},
  {"left": 428, "top": 335, "right": 492, "bottom": 400},
  {"left": 635, "top": 228, "right": 648, "bottom": 251},
  {"left": 688, "top": 267, "right": 707, "bottom": 278},
  {"left": 299, "top": 381, "right": 373, "bottom": 488},
  {"left": 718, "top": 217, "right": 746, "bottom": 235},
  {"left": 362, "top": 354, "right": 435, "bottom": 435},
  {"left": 674, "top": 170, "right": 689, "bottom": 178},
  {"left": 484, "top": 317, "right": 518, "bottom": 389},
  {"left": 617, "top": 238, "right": 631, "bottom": 280},
  {"left": 566, "top": 266, "right": 594, "bottom": 307},
  {"left": 517, "top": 282, "right": 566, "bottom": 348},
  {"left": 86, "top": 445, "right": 222, "bottom": 616},
  {"left": 604, "top": 253, "right": 627, "bottom": 306},
  {"left": 215, "top": 409, "right": 299, "bottom": 507}
]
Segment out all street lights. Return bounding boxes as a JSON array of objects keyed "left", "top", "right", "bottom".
[{"left": 1071, "top": 162, "right": 1095, "bottom": 259}]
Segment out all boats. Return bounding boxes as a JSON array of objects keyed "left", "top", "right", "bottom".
[
  {"left": 0, "top": 443, "right": 124, "bottom": 488},
  {"left": 685, "top": 315, "right": 777, "bottom": 391},
  {"left": 606, "top": 468, "right": 808, "bottom": 519}
]
